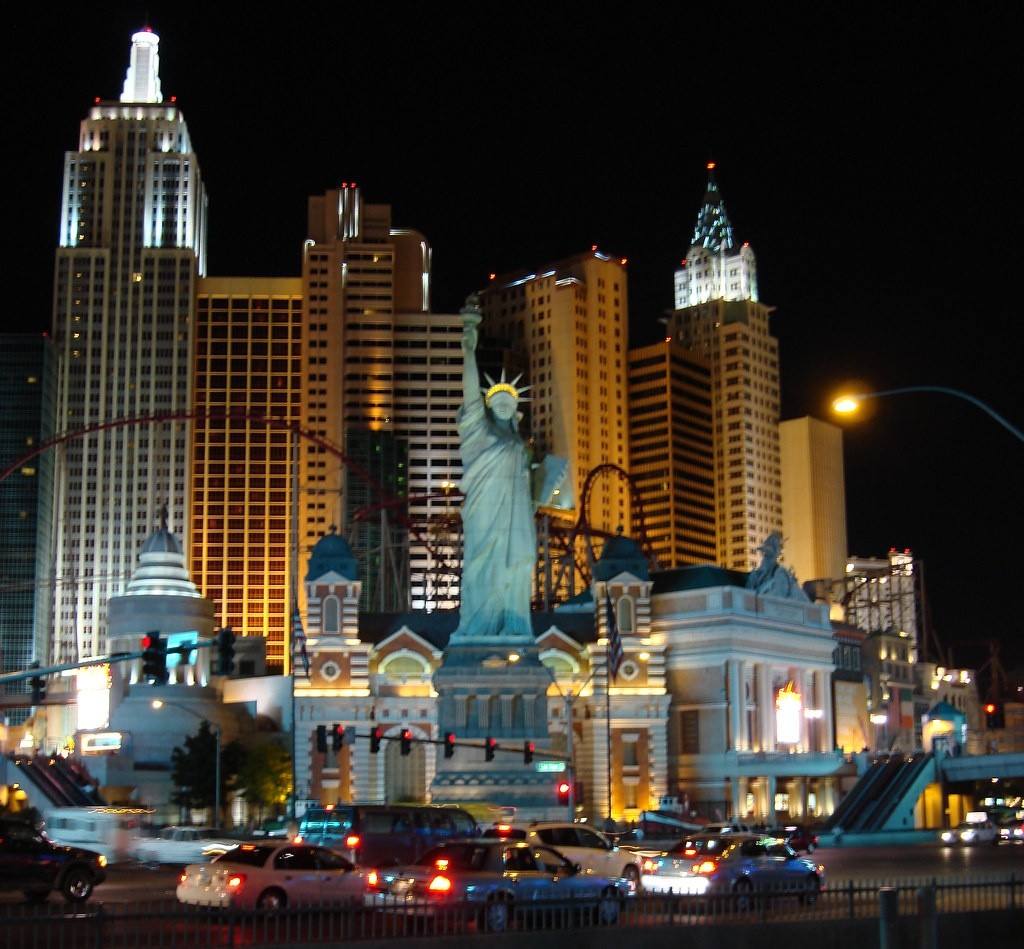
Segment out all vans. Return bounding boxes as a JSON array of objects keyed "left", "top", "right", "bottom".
[
  {"left": 293, "top": 804, "right": 484, "bottom": 870},
  {"left": 40, "top": 806, "right": 143, "bottom": 866}
]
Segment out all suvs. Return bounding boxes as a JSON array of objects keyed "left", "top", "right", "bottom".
[{"left": 481, "top": 818, "right": 646, "bottom": 895}]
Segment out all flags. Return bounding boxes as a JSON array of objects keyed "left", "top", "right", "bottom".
[
  {"left": 292, "top": 591, "right": 310, "bottom": 678},
  {"left": 607, "top": 595, "right": 624, "bottom": 682}
]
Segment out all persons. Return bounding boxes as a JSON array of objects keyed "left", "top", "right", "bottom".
[
  {"left": 313, "top": 849, "right": 343, "bottom": 870},
  {"left": 985, "top": 739, "right": 998, "bottom": 754},
  {"left": 514, "top": 851, "right": 532, "bottom": 870},
  {"left": 442, "top": 809, "right": 460, "bottom": 829},
  {"left": 953, "top": 742, "right": 962, "bottom": 757},
  {"left": 450, "top": 305, "right": 567, "bottom": 638},
  {"left": 414, "top": 812, "right": 430, "bottom": 826}
]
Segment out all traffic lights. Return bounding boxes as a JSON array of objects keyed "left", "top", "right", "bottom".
[
  {"left": 371, "top": 726, "right": 383, "bottom": 753},
  {"left": 215, "top": 627, "right": 236, "bottom": 676},
  {"left": 985, "top": 701, "right": 997, "bottom": 729},
  {"left": 400, "top": 728, "right": 412, "bottom": 755},
  {"left": 524, "top": 742, "right": 534, "bottom": 763},
  {"left": 445, "top": 732, "right": 456, "bottom": 758},
  {"left": 26, "top": 661, "right": 48, "bottom": 703},
  {"left": 485, "top": 735, "right": 497, "bottom": 762},
  {"left": 558, "top": 782, "right": 569, "bottom": 806},
  {"left": 333, "top": 722, "right": 344, "bottom": 751},
  {"left": 142, "top": 630, "right": 162, "bottom": 680}
]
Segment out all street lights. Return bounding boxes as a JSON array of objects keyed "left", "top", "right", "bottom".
[{"left": 506, "top": 650, "right": 652, "bottom": 821}]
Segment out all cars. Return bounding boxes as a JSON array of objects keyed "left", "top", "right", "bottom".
[
  {"left": 175, "top": 842, "right": 368, "bottom": 916},
  {"left": 615, "top": 822, "right": 824, "bottom": 911},
  {"left": 0, "top": 816, "right": 109, "bottom": 907},
  {"left": 132, "top": 827, "right": 245, "bottom": 866},
  {"left": 362, "top": 835, "right": 630, "bottom": 934},
  {"left": 938, "top": 805, "right": 1024, "bottom": 846}
]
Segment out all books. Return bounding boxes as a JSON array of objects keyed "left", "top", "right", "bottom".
[{"left": 531, "top": 454, "right": 570, "bottom": 507}]
